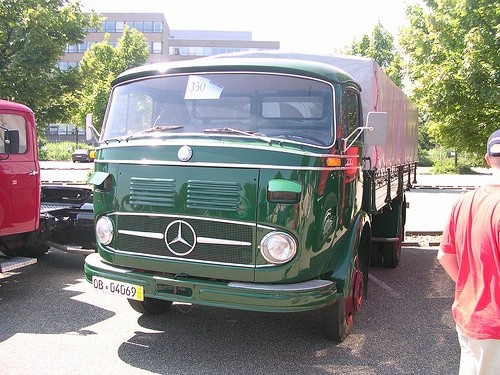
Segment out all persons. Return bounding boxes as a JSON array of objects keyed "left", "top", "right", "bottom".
[{"left": 436, "top": 130, "right": 500, "bottom": 375}]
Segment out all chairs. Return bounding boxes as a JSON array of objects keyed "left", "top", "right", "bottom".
[{"left": 153, "top": 102, "right": 203, "bottom": 135}]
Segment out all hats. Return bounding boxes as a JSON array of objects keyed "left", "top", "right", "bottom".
[{"left": 487, "top": 129, "right": 500, "bottom": 157}]
